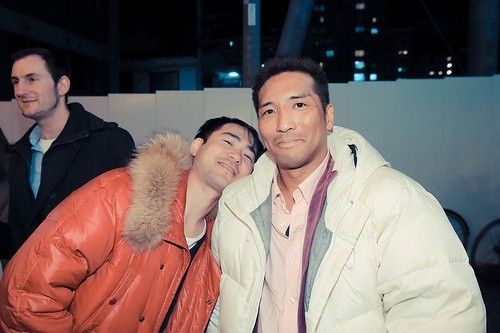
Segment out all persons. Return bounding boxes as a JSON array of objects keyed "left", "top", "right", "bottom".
[
  {"left": 204, "top": 56, "right": 487, "bottom": 333},
  {"left": 6, "top": 46, "right": 139, "bottom": 259},
  {"left": 0, "top": 116, "right": 264, "bottom": 333}
]
